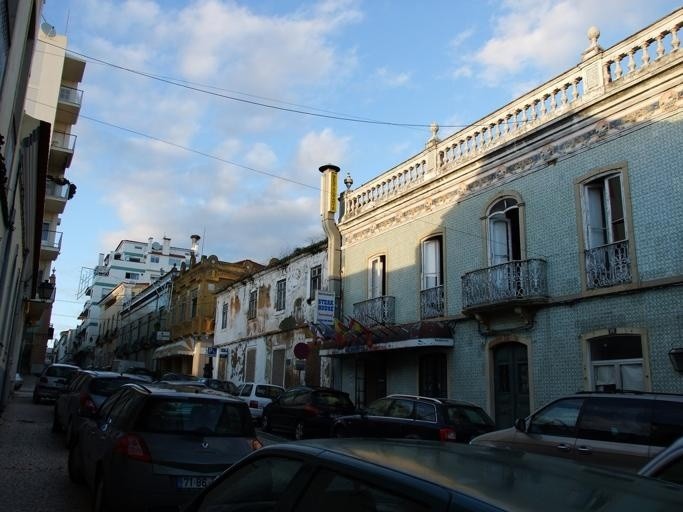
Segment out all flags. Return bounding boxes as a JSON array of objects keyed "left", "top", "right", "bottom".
[{"left": 306, "top": 318, "right": 366, "bottom": 346}]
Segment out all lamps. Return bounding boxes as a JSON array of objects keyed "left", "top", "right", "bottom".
[
  {"left": 24, "top": 269, "right": 55, "bottom": 301},
  {"left": 667, "top": 346, "right": 683, "bottom": 375}
]
